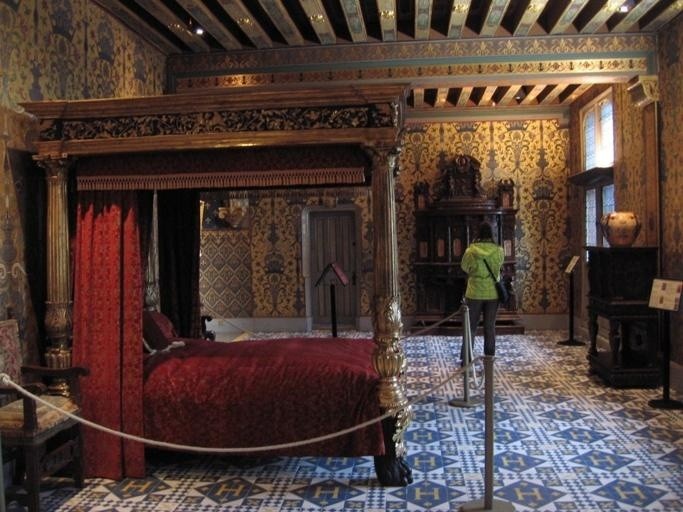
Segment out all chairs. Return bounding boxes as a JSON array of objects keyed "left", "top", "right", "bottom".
[{"left": 1, "top": 305, "right": 88, "bottom": 512}]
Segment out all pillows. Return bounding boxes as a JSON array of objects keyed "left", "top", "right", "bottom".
[{"left": 143, "top": 303, "right": 187, "bottom": 356}]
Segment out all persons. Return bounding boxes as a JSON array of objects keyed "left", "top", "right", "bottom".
[{"left": 458, "top": 222, "right": 506, "bottom": 367}]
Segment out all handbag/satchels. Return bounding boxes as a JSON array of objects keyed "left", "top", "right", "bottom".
[{"left": 496, "top": 281, "right": 510, "bottom": 303}]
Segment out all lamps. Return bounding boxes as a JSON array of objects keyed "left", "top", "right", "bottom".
[{"left": 164, "top": 14, "right": 206, "bottom": 39}]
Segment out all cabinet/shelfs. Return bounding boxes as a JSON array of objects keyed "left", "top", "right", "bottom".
[
  {"left": 579, "top": 244, "right": 666, "bottom": 389},
  {"left": 409, "top": 154, "right": 525, "bottom": 335}
]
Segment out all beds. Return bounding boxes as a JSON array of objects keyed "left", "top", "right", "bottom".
[{"left": 16, "top": 80, "right": 416, "bottom": 487}]
